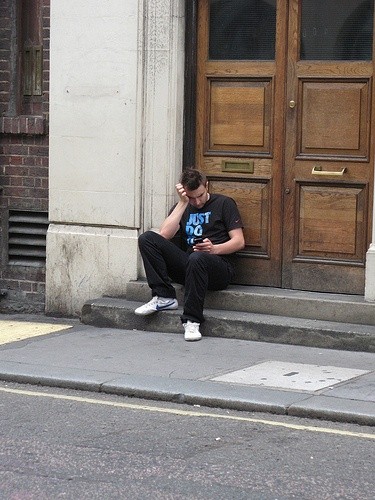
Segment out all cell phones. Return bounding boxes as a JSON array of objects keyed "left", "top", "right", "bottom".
[{"left": 194, "top": 238, "right": 203, "bottom": 244}]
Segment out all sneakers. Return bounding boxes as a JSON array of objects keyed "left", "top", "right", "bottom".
[
  {"left": 135, "top": 295, "right": 179, "bottom": 316},
  {"left": 183, "top": 319, "right": 202, "bottom": 341}
]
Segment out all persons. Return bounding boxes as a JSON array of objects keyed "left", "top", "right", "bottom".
[{"left": 134, "top": 168, "right": 246, "bottom": 341}]
